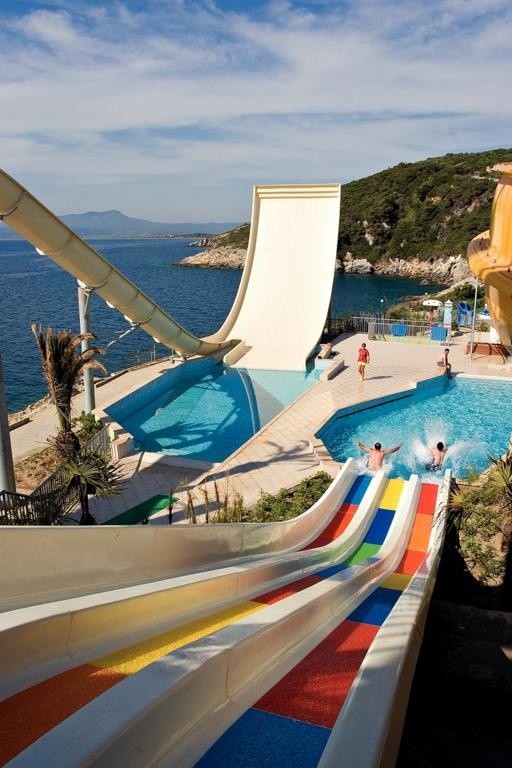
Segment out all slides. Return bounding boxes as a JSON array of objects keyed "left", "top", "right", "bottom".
[
  {"left": 2, "top": 456, "right": 451, "bottom": 763},
  {"left": 1, "top": 168, "right": 340, "bottom": 373}
]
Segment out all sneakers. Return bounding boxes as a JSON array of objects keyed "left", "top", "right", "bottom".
[{"left": 360, "top": 376, "right": 364, "bottom": 381}]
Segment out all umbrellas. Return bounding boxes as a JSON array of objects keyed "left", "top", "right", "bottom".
[{"left": 421, "top": 299, "right": 443, "bottom": 308}]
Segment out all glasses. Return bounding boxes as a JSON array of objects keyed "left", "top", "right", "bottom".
[{"left": 362, "top": 346, "right": 365, "bottom": 347}]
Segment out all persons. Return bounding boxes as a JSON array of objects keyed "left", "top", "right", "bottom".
[
  {"left": 317, "top": 342, "right": 339, "bottom": 359},
  {"left": 425, "top": 442, "right": 448, "bottom": 472},
  {"left": 357, "top": 441, "right": 406, "bottom": 474},
  {"left": 356, "top": 343, "right": 370, "bottom": 382},
  {"left": 435, "top": 348, "right": 452, "bottom": 378},
  {"left": 425, "top": 306, "right": 435, "bottom": 329}
]
